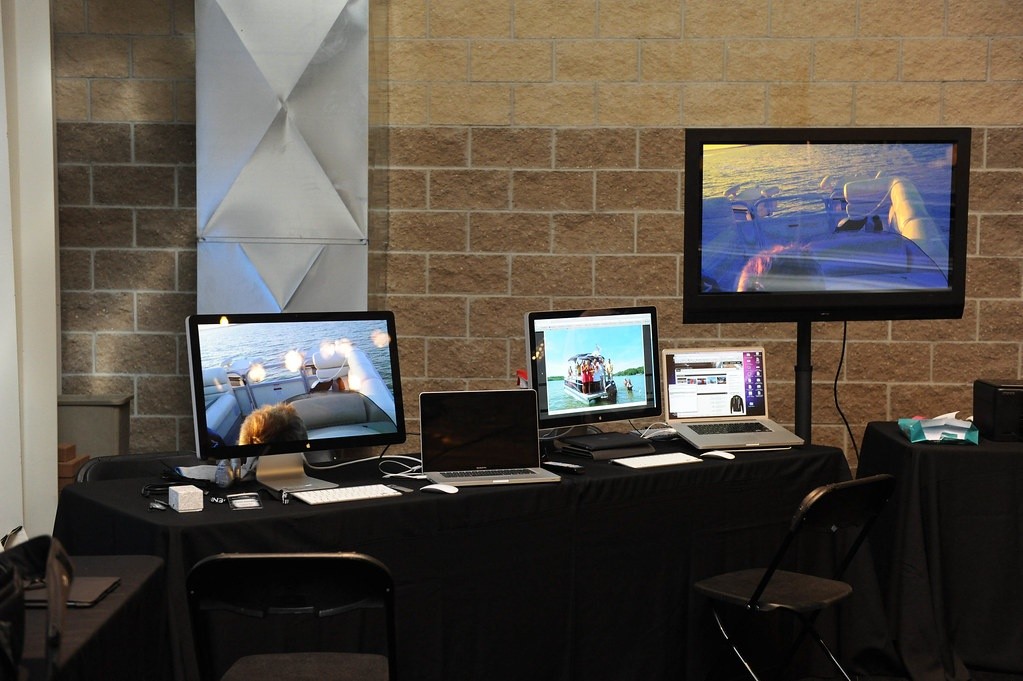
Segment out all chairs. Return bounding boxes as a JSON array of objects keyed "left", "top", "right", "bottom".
[
  {"left": 691, "top": 475, "right": 899, "bottom": 681},
  {"left": 185, "top": 552, "right": 396, "bottom": 681}
]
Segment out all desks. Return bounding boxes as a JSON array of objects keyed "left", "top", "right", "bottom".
[
  {"left": 61, "top": 425, "right": 853, "bottom": 681},
  {"left": 860, "top": 422, "right": 1023, "bottom": 681},
  {"left": 21, "top": 554, "right": 173, "bottom": 681}
]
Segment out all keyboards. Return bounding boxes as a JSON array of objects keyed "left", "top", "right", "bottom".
[
  {"left": 610, "top": 451, "right": 704, "bottom": 469},
  {"left": 289, "top": 484, "right": 403, "bottom": 507}
]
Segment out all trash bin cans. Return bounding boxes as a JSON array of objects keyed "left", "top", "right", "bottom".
[{"left": 57, "top": 394, "right": 134, "bottom": 455}]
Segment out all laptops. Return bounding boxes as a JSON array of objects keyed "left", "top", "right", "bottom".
[
  {"left": 661, "top": 346, "right": 805, "bottom": 449},
  {"left": 419, "top": 389, "right": 562, "bottom": 486}
]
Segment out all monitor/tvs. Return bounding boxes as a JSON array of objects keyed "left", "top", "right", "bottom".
[
  {"left": 185, "top": 310, "right": 406, "bottom": 460},
  {"left": 682, "top": 127, "right": 972, "bottom": 324},
  {"left": 524, "top": 306, "right": 663, "bottom": 448}
]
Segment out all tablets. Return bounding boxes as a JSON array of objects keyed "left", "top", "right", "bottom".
[
  {"left": 559, "top": 431, "right": 651, "bottom": 451},
  {"left": 22, "top": 577, "right": 122, "bottom": 608}
]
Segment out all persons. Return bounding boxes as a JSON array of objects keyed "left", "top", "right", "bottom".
[
  {"left": 737, "top": 244, "right": 827, "bottom": 291},
  {"left": 624, "top": 378, "right": 631, "bottom": 386},
  {"left": 238, "top": 400, "right": 308, "bottom": 445},
  {"left": 569, "top": 358, "right": 613, "bottom": 394}
]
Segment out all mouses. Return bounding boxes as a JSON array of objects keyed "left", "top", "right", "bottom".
[
  {"left": 700, "top": 450, "right": 736, "bottom": 459},
  {"left": 419, "top": 485, "right": 459, "bottom": 494}
]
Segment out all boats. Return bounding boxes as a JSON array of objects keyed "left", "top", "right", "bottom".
[
  {"left": 562, "top": 343, "right": 618, "bottom": 405},
  {"left": 703, "top": 169, "right": 948, "bottom": 293},
  {"left": 202, "top": 337, "right": 398, "bottom": 446}
]
